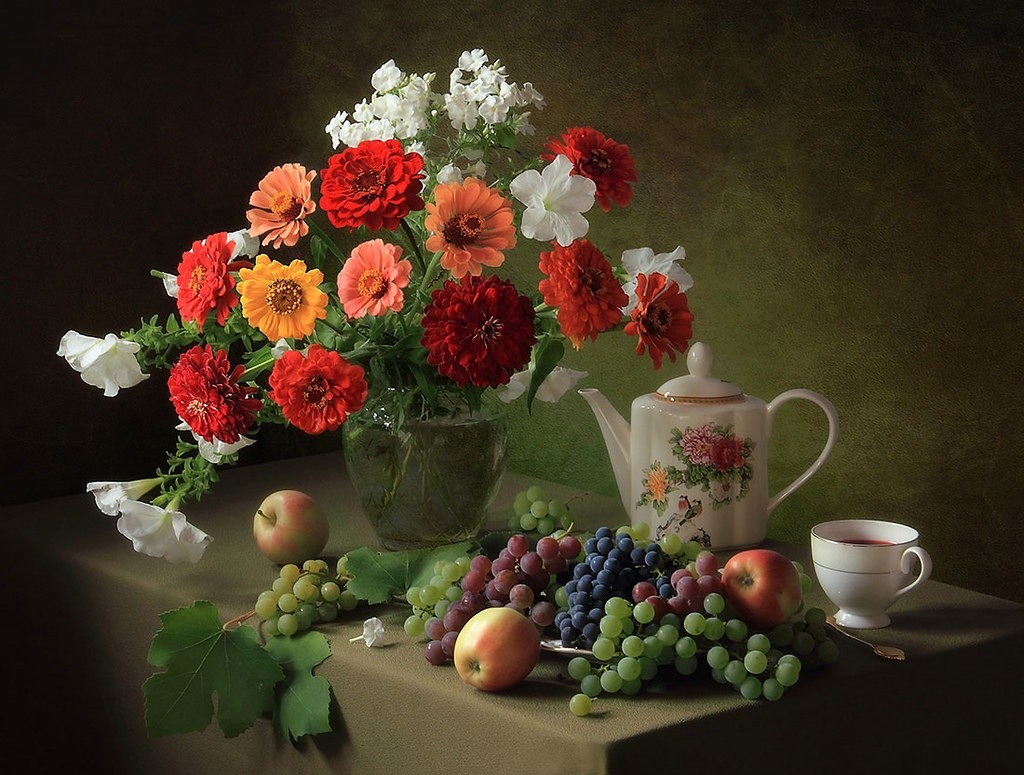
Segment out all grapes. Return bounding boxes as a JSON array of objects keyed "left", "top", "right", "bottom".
[
  {"left": 255, "top": 557, "right": 359, "bottom": 635},
  {"left": 404, "top": 486, "right": 838, "bottom": 716}
]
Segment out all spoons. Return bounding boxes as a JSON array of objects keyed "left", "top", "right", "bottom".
[{"left": 826, "top": 616, "right": 905, "bottom": 661}]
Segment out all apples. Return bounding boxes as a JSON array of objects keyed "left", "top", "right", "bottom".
[
  {"left": 253, "top": 490, "right": 330, "bottom": 564},
  {"left": 722, "top": 550, "right": 801, "bottom": 629},
  {"left": 453, "top": 607, "right": 540, "bottom": 691}
]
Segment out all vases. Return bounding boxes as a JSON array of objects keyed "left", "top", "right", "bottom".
[{"left": 340, "top": 390, "right": 513, "bottom": 552}]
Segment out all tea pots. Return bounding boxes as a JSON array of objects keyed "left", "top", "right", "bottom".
[{"left": 577, "top": 341, "right": 838, "bottom": 552}]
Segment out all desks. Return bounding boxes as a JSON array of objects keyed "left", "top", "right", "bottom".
[{"left": 0, "top": 451, "right": 1024, "bottom": 775}]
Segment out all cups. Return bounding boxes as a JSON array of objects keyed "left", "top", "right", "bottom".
[{"left": 809, "top": 517, "right": 932, "bottom": 630}]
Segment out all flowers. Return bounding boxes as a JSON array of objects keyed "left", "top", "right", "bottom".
[{"left": 54, "top": 47, "right": 694, "bottom": 561}]
